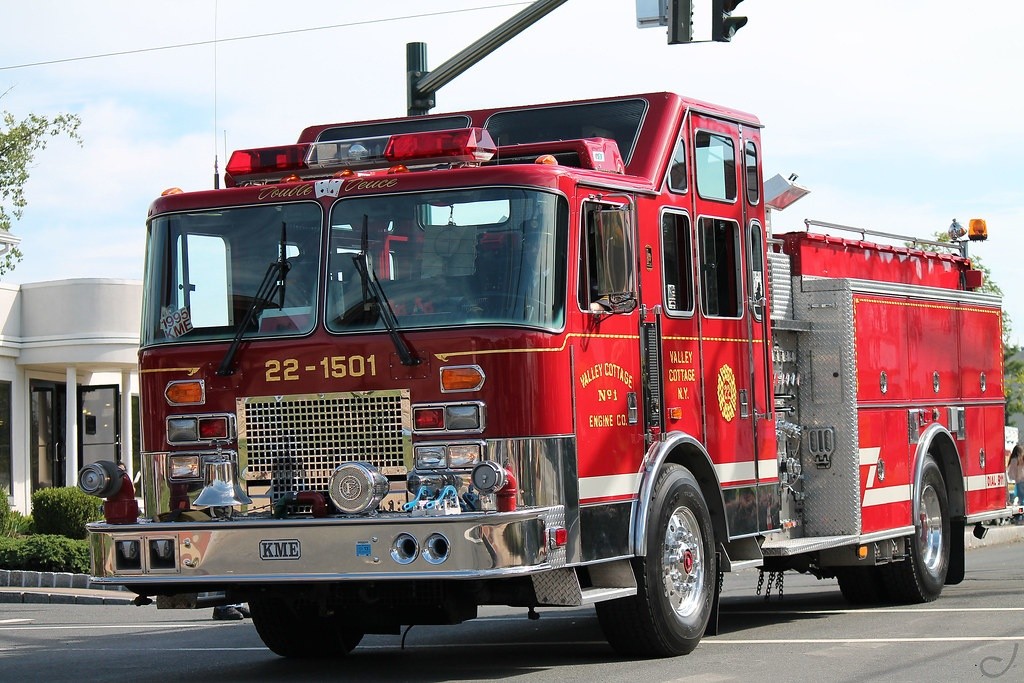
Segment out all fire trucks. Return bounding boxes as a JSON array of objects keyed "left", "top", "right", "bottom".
[{"left": 77, "top": 91, "right": 1024, "bottom": 663}]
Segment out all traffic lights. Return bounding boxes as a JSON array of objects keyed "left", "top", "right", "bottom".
[{"left": 712, "top": 0, "right": 748, "bottom": 42}]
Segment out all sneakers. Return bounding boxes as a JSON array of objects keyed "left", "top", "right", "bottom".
[
  {"left": 236, "top": 606, "right": 252, "bottom": 618},
  {"left": 213, "top": 606, "right": 244, "bottom": 620}
]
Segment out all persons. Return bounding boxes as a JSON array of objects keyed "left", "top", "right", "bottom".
[
  {"left": 213, "top": 603, "right": 251, "bottom": 620},
  {"left": 1007, "top": 445, "right": 1024, "bottom": 525},
  {"left": 488, "top": 217, "right": 610, "bottom": 326}
]
